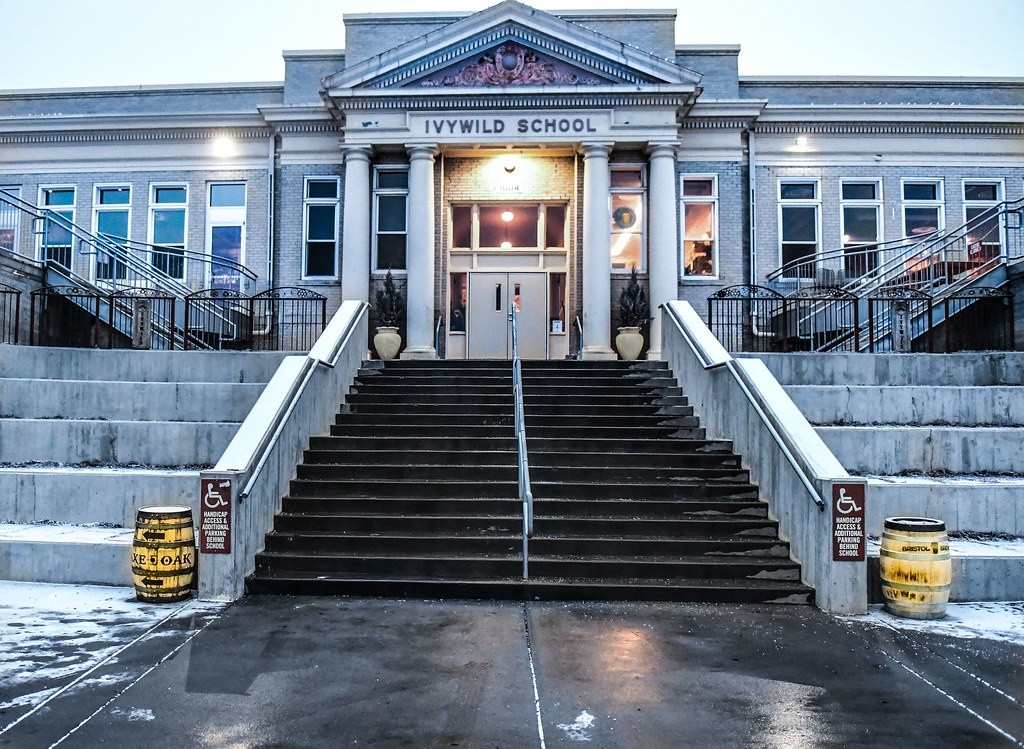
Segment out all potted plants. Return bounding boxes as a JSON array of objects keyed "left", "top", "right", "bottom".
[
  {"left": 613, "top": 265, "right": 654, "bottom": 361},
  {"left": 372, "top": 262, "right": 405, "bottom": 361}
]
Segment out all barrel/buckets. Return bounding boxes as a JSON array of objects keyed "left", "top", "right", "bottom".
[
  {"left": 880, "top": 517, "right": 952, "bottom": 621},
  {"left": 131, "top": 505, "right": 195, "bottom": 603}
]
